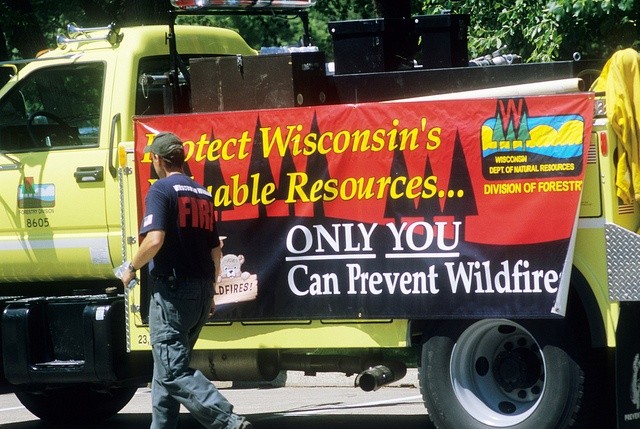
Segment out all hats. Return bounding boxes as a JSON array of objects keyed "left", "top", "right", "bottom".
[{"left": 144, "top": 132, "right": 184, "bottom": 164}]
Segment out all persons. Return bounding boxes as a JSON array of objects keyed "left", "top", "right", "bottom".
[{"left": 121, "top": 130, "right": 253, "bottom": 428}]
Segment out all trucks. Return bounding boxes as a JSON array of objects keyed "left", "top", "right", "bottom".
[{"left": 2, "top": 0, "right": 640, "bottom": 428}]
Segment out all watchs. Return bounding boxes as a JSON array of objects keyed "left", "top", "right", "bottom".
[{"left": 128, "top": 263, "right": 136, "bottom": 272}]
